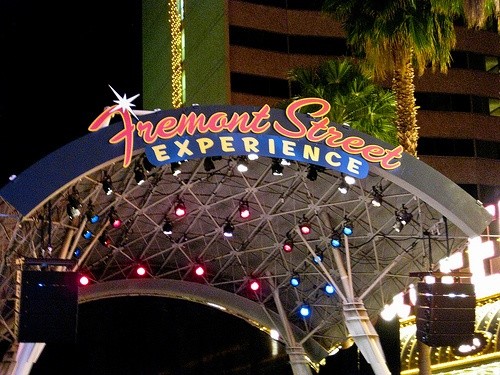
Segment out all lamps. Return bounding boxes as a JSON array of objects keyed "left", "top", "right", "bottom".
[
  {"left": 391, "top": 204, "right": 413, "bottom": 232},
  {"left": 36, "top": 156, "right": 354, "bottom": 317},
  {"left": 368, "top": 188, "right": 385, "bottom": 209}
]
